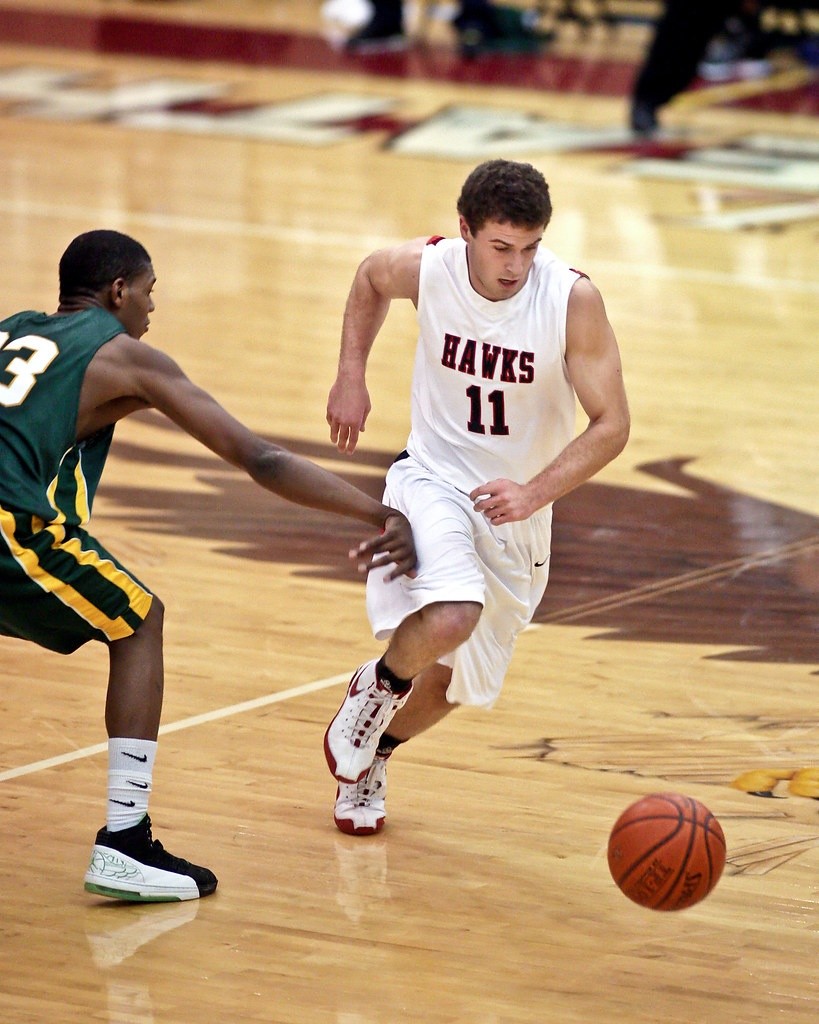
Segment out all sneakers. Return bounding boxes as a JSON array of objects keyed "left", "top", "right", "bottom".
[
  {"left": 324, "top": 656, "right": 414, "bottom": 784},
  {"left": 84, "top": 812, "right": 218, "bottom": 901},
  {"left": 334, "top": 752, "right": 392, "bottom": 835}
]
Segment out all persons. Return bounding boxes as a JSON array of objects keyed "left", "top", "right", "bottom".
[
  {"left": 342, "top": 0, "right": 819, "bottom": 144},
  {"left": 0, "top": 230, "right": 419, "bottom": 904},
  {"left": 324, "top": 159, "right": 631, "bottom": 835}
]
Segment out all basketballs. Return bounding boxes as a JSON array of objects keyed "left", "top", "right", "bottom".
[{"left": 607, "top": 792, "right": 727, "bottom": 912}]
